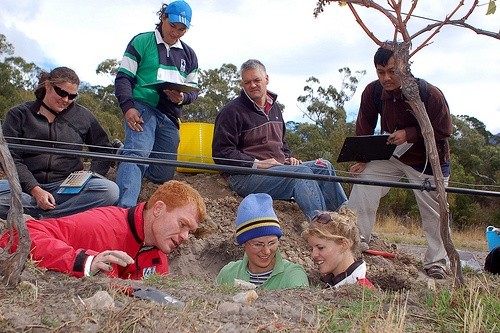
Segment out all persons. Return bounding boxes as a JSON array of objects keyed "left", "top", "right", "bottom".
[
  {"left": 347, "top": 40, "right": 451, "bottom": 279},
  {"left": 0, "top": 179, "right": 208, "bottom": 280},
  {"left": 211, "top": 58, "right": 348, "bottom": 224},
  {"left": 0, "top": 66, "right": 121, "bottom": 222},
  {"left": 213, "top": 193, "right": 309, "bottom": 292},
  {"left": 300, "top": 204, "right": 378, "bottom": 294},
  {"left": 108, "top": 0, "right": 201, "bottom": 209}
]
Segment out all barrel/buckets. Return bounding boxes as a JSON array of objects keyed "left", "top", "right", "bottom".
[
  {"left": 176, "top": 117, "right": 219, "bottom": 173},
  {"left": 486, "top": 226, "right": 500, "bottom": 253}
]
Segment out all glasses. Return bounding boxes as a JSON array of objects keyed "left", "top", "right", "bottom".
[
  {"left": 247, "top": 237, "right": 279, "bottom": 248},
  {"left": 311, "top": 213, "right": 334, "bottom": 223},
  {"left": 51, "top": 82, "right": 79, "bottom": 99}
]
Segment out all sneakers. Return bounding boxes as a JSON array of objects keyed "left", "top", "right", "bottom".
[
  {"left": 427, "top": 264, "right": 446, "bottom": 279},
  {"left": 109, "top": 137, "right": 123, "bottom": 169}
]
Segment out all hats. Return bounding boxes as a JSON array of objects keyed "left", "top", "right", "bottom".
[
  {"left": 165, "top": 0, "right": 193, "bottom": 28},
  {"left": 235, "top": 192, "right": 282, "bottom": 243}
]
